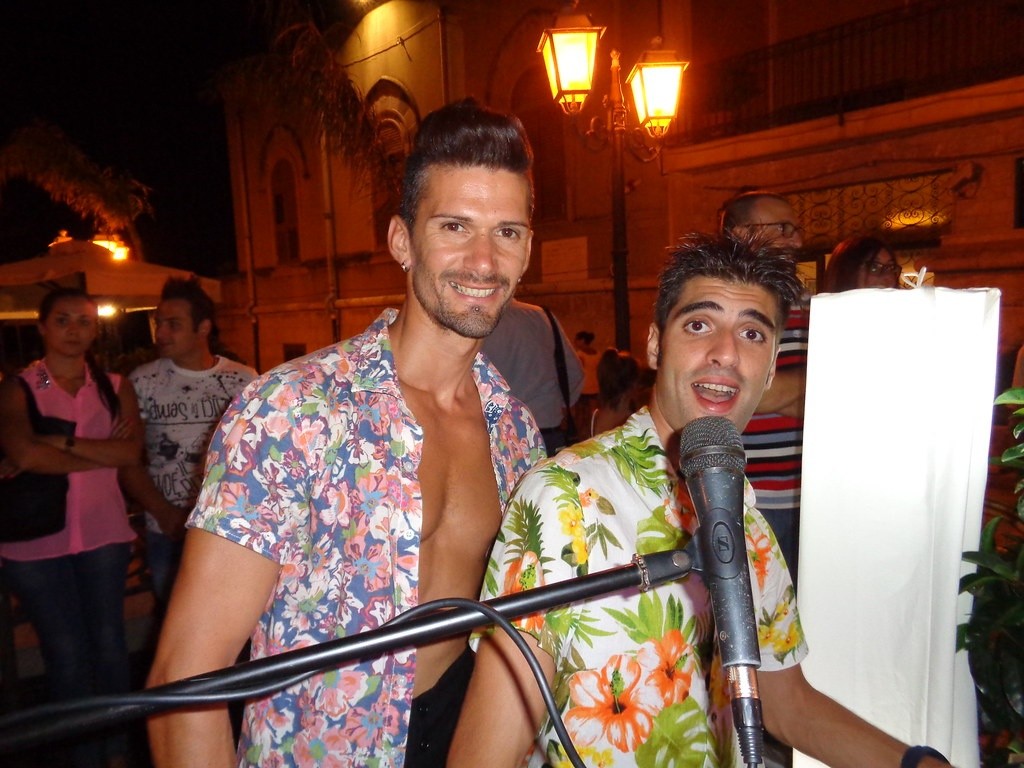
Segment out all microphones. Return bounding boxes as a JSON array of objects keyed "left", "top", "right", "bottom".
[{"left": 679, "top": 416, "right": 765, "bottom": 763}]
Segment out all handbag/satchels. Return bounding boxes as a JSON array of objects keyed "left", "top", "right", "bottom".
[{"left": 0, "top": 376, "right": 77, "bottom": 543}]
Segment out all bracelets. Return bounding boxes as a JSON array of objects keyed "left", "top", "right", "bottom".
[{"left": 900, "top": 745, "right": 950, "bottom": 768}]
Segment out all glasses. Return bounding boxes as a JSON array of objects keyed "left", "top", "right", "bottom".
[
  {"left": 861, "top": 261, "right": 903, "bottom": 278},
  {"left": 748, "top": 221, "right": 806, "bottom": 240}
]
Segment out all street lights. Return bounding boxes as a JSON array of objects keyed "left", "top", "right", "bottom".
[{"left": 534, "top": 4, "right": 692, "bottom": 353}]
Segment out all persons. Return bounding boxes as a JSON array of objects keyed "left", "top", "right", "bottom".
[
  {"left": 147, "top": 96, "right": 546, "bottom": 768},
  {"left": 0, "top": 286, "right": 147, "bottom": 704},
  {"left": 572, "top": 329, "right": 658, "bottom": 446},
  {"left": 447, "top": 225, "right": 954, "bottom": 768},
  {"left": 721, "top": 192, "right": 810, "bottom": 591},
  {"left": 480, "top": 291, "right": 583, "bottom": 457},
  {"left": 125, "top": 280, "right": 258, "bottom": 629},
  {"left": 823, "top": 236, "right": 901, "bottom": 294}
]
